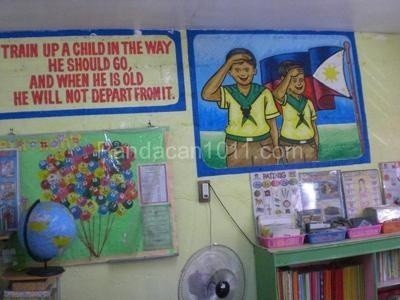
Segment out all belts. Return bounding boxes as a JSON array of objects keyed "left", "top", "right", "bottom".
[
  {"left": 280, "top": 134, "right": 315, "bottom": 144},
  {"left": 225, "top": 133, "right": 271, "bottom": 143}
]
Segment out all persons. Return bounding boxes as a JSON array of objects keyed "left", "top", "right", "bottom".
[{"left": 201, "top": 48, "right": 319, "bottom": 168}]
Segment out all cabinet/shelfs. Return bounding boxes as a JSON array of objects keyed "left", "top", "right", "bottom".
[{"left": 253, "top": 233, "right": 400, "bottom": 299}]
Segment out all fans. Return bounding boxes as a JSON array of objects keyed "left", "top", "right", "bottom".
[{"left": 178, "top": 243, "right": 246, "bottom": 300}]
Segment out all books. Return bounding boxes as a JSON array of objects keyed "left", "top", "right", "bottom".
[
  {"left": 2, "top": 278, "right": 57, "bottom": 300},
  {"left": 276, "top": 249, "right": 400, "bottom": 300}
]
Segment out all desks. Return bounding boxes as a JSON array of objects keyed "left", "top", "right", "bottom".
[{"left": 0, "top": 265, "right": 65, "bottom": 299}]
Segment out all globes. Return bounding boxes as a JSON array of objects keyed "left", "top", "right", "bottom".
[{"left": 19, "top": 199, "right": 77, "bottom": 277}]
